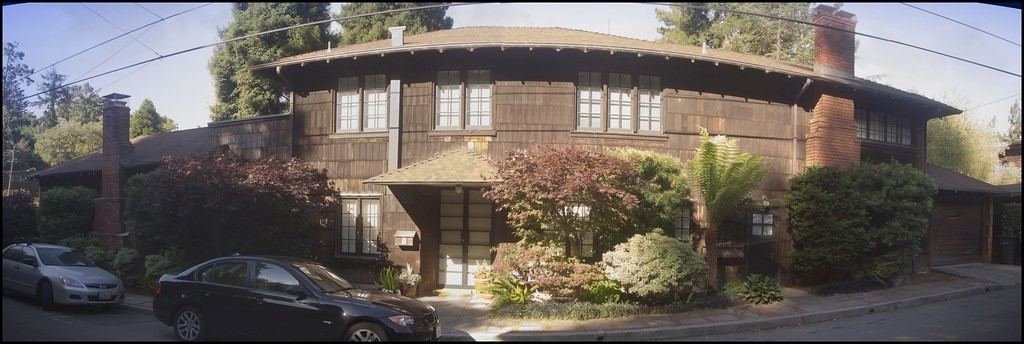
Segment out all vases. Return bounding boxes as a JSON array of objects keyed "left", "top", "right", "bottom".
[{"left": 398, "top": 262, "right": 422, "bottom": 298}]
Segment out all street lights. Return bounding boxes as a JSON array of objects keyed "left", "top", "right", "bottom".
[{"left": 7, "top": 150, "right": 14, "bottom": 196}]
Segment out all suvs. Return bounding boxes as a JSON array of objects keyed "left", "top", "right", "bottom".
[{"left": 2, "top": 241, "right": 125, "bottom": 311}]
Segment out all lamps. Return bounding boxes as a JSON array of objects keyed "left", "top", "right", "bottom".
[{"left": 455, "top": 184, "right": 462, "bottom": 193}]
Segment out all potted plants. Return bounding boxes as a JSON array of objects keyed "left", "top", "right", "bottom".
[
  {"left": 479, "top": 287, "right": 495, "bottom": 300},
  {"left": 701, "top": 240, "right": 744, "bottom": 258},
  {"left": 374, "top": 265, "right": 401, "bottom": 295},
  {"left": 473, "top": 259, "right": 494, "bottom": 292}
]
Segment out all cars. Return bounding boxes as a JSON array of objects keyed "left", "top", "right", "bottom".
[{"left": 152, "top": 256, "right": 441, "bottom": 344}]
